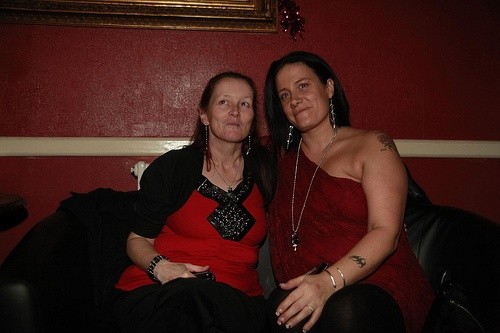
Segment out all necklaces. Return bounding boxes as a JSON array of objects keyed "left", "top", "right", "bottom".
[
  {"left": 292, "top": 130, "right": 336, "bottom": 251},
  {"left": 209, "top": 157, "right": 241, "bottom": 195}
]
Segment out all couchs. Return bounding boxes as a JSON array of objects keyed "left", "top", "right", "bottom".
[{"left": 1, "top": 188, "right": 500, "bottom": 332}]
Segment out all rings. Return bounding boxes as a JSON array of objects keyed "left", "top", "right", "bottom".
[{"left": 308, "top": 304, "right": 315, "bottom": 311}]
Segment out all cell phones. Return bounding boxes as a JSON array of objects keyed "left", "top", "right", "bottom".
[{"left": 193, "top": 272, "right": 216, "bottom": 282}]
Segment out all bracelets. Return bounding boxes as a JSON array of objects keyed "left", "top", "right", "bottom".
[
  {"left": 147, "top": 254, "right": 170, "bottom": 281},
  {"left": 324, "top": 268, "right": 346, "bottom": 288}
]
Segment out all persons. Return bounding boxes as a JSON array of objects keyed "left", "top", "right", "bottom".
[
  {"left": 109, "top": 70, "right": 277, "bottom": 333},
  {"left": 255, "top": 51, "right": 431, "bottom": 333}
]
